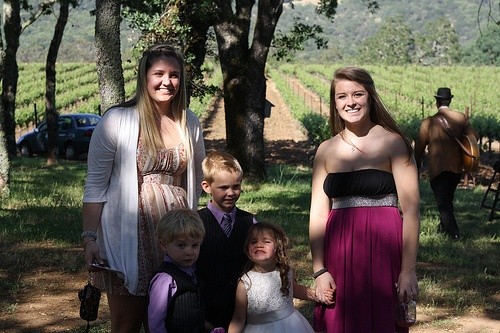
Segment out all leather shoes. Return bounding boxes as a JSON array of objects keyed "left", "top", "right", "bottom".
[
  {"left": 454, "top": 234, "right": 461, "bottom": 240},
  {"left": 437, "top": 224, "right": 444, "bottom": 232}
]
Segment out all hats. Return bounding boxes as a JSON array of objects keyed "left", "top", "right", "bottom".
[{"left": 435, "top": 87, "right": 454, "bottom": 99}]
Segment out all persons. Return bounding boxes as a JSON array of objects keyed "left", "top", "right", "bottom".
[
  {"left": 192, "top": 149, "right": 259, "bottom": 332},
  {"left": 81, "top": 44, "right": 209, "bottom": 332},
  {"left": 311, "top": 67, "right": 422, "bottom": 333},
  {"left": 414, "top": 86, "right": 473, "bottom": 241},
  {"left": 146, "top": 208, "right": 227, "bottom": 333},
  {"left": 227, "top": 222, "right": 335, "bottom": 333}
]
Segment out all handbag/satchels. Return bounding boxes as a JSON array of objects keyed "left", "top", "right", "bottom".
[{"left": 461, "top": 135, "right": 479, "bottom": 173}]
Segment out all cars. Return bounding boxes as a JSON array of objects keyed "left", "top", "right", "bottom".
[{"left": 18, "top": 114, "right": 102, "bottom": 160}]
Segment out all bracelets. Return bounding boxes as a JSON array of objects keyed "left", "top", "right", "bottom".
[
  {"left": 312, "top": 268, "right": 328, "bottom": 278},
  {"left": 80, "top": 230, "right": 98, "bottom": 248}
]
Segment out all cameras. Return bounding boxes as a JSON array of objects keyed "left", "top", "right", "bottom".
[{"left": 77, "top": 284, "right": 101, "bottom": 322}]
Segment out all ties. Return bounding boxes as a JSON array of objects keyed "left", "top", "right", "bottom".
[
  {"left": 191, "top": 272, "right": 198, "bottom": 287},
  {"left": 223, "top": 215, "right": 232, "bottom": 237}
]
